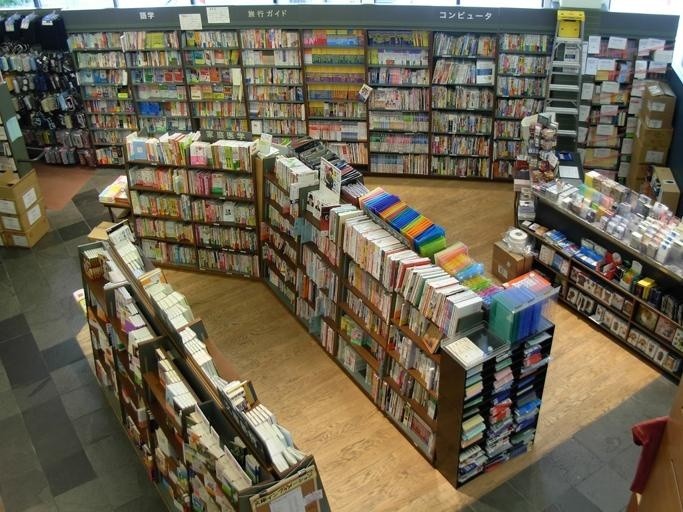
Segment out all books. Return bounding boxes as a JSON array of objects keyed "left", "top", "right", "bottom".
[
  {"left": 72, "top": 222, "right": 306, "bottom": 511},
  {"left": 430, "top": 32, "right": 494, "bottom": 177},
  {"left": 302, "top": 27, "right": 367, "bottom": 163},
  {"left": 239, "top": 27, "right": 307, "bottom": 144},
  {"left": 126, "top": 130, "right": 262, "bottom": 278},
  {"left": 490, "top": 31, "right": 546, "bottom": 181},
  {"left": 179, "top": 27, "right": 246, "bottom": 129},
  {"left": 261, "top": 136, "right": 543, "bottom": 483},
  {"left": 99, "top": 175, "right": 131, "bottom": 206},
  {"left": 69, "top": 29, "right": 191, "bottom": 165},
  {"left": 365, "top": 28, "right": 429, "bottom": 174}
]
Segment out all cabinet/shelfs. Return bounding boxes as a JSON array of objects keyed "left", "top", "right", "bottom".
[
  {"left": 622, "top": 375, "right": 682, "bottom": 511},
  {"left": 0, "top": 9, "right": 91, "bottom": 170},
  {"left": 498, "top": 181, "right": 683, "bottom": 391},
  {"left": 1, "top": 71, "right": 50, "bottom": 248},
  {"left": 74, "top": 215, "right": 332, "bottom": 510},
  {"left": 98, "top": 132, "right": 555, "bottom": 491},
  {"left": 60, "top": 8, "right": 682, "bottom": 180}
]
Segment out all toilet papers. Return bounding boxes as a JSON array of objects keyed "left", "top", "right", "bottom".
[{"left": 508, "top": 229, "right": 528, "bottom": 254}]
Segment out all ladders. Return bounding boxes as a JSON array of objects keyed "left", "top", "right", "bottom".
[{"left": 538, "top": 10, "right": 586, "bottom": 151}]
